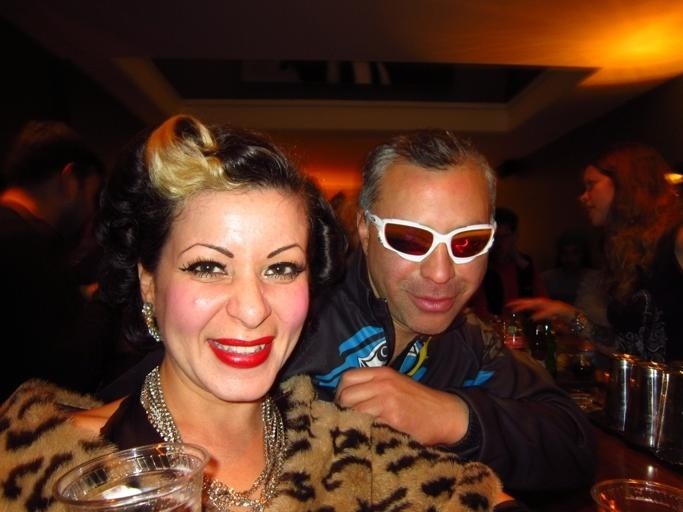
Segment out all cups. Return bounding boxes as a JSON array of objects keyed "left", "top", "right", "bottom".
[
  {"left": 54, "top": 441, "right": 208, "bottom": 512},
  {"left": 592, "top": 477, "right": 682, "bottom": 512}
]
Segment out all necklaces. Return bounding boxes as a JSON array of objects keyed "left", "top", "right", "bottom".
[{"left": 138, "top": 363, "right": 288, "bottom": 512}]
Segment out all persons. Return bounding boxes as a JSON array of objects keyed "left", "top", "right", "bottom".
[
  {"left": 97, "top": 129, "right": 598, "bottom": 511},
  {"left": 1, "top": 119, "right": 116, "bottom": 394},
  {"left": 480, "top": 207, "right": 538, "bottom": 357},
  {"left": 1, "top": 112, "right": 519, "bottom": 511},
  {"left": 506, "top": 137, "right": 682, "bottom": 364}
]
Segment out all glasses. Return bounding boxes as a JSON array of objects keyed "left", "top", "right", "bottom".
[{"left": 359, "top": 204, "right": 498, "bottom": 266}]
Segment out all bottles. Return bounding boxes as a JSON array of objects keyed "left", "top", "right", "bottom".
[{"left": 540, "top": 319, "right": 558, "bottom": 374}]
